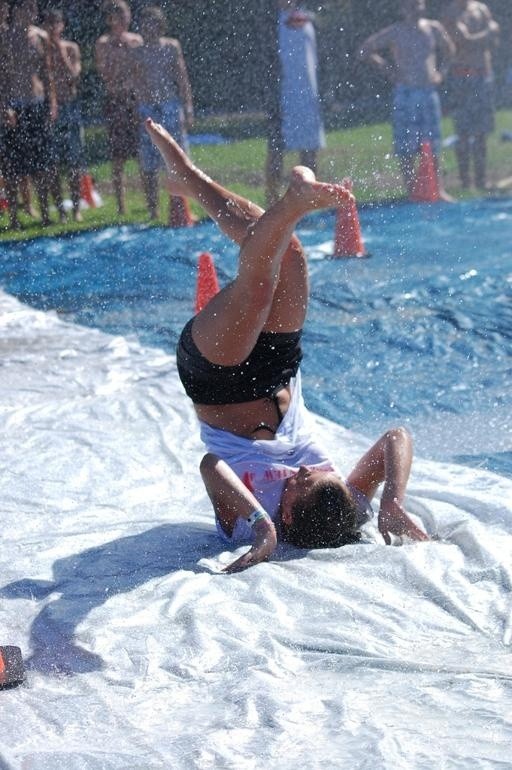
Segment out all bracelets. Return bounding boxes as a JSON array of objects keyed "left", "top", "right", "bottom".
[{"left": 246, "top": 507, "right": 267, "bottom": 528}]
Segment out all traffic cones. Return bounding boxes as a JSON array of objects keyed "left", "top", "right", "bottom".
[
  {"left": 1, "top": 176, "right": 9, "bottom": 208},
  {"left": 405, "top": 140, "right": 439, "bottom": 203},
  {"left": 335, "top": 178, "right": 365, "bottom": 258},
  {"left": 195, "top": 252, "right": 220, "bottom": 314},
  {"left": 169, "top": 193, "right": 194, "bottom": 228},
  {"left": 78, "top": 170, "right": 102, "bottom": 210}
]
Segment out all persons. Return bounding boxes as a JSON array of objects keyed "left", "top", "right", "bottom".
[
  {"left": 94, "top": 0, "right": 192, "bottom": 222},
  {"left": 355, "top": 1, "right": 457, "bottom": 203},
  {"left": 438, "top": 0, "right": 502, "bottom": 189},
  {"left": 1, "top": 0, "right": 82, "bottom": 232},
  {"left": 141, "top": 114, "right": 431, "bottom": 574},
  {"left": 277, "top": 0, "right": 327, "bottom": 176}
]
